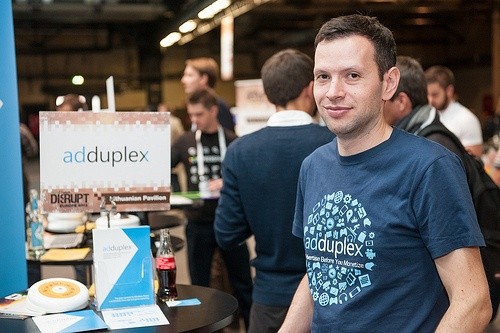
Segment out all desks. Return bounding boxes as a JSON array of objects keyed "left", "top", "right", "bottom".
[{"left": 0, "top": 215, "right": 240, "bottom": 333}]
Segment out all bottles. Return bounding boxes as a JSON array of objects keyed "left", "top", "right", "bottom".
[
  {"left": 27, "top": 190, "right": 45, "bottom": 255},
  {"left": 156, "top": 229, "right": 178, "bottom": 299}
]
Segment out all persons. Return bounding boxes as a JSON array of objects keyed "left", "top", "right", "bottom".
[
  {"left": 383, "top": 55, "right": 476, "bottom": 195},
  {"left": 212, "top": 48, "right": 336, "bottom": 333},
  {"left": 278, "top": 15, "right": 494, "bottom": 333},
  {"left": 157, "top": 58, "right": 236, "bottom": 133},
  {"left": 424, "top": 65, "right": 483, "bottom": 160},
  {"left": 170, "top": 87, "right": 253, "bottom": 333},
  {"left": 55, "top": 94, "right": 89, "bottom": 111}
]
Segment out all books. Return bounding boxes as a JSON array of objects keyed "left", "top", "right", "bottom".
[{"left": 92, "top": 226, "right": 155, "bottom": 311}]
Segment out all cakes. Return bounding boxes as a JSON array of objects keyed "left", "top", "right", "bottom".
[
  {"left": 28, "top": 278, "right": 89, "bottom": 313},
  {"left": 45, "top": 213, "right": 85, "bottom": 234},
  {"left": 95, "top": 214, "right": 139, "bottom": 227}
]
